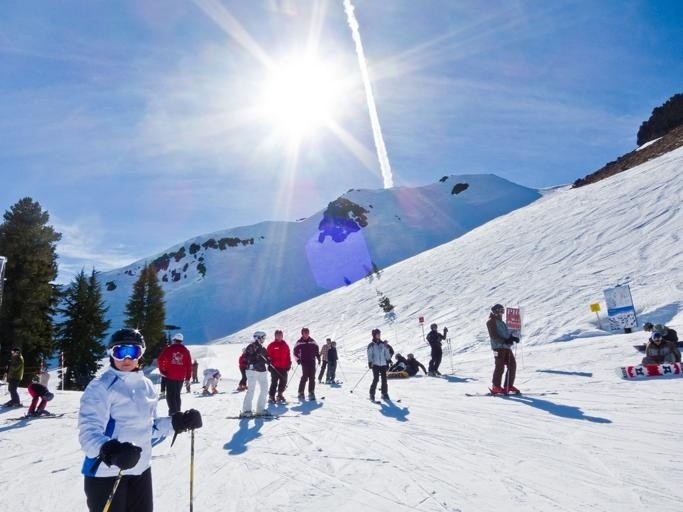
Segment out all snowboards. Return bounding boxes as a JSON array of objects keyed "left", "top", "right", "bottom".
[
  {"left": 633, "top": 341, "right": 683, "bottom": 352},
  {"left": 614, "top": 362, "right": 683, "bottom": 379},
  {"left": 386, "top": 371, "right": 408, "bottom": 377}
]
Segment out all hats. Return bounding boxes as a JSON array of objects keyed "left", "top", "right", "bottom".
[
  {"left": 46, "top": 392, "right": 54, "bottom": 401},
  {"left": 172, "top": 333, "right": 184, "bottom": 341}
]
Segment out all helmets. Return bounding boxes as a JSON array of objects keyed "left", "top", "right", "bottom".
[
  {"left": 491, "top": 304, "right": 504, "bottom": 315},
  {"left": 643, "top": 322, "right": 664, "bottom": 341},
  {"left": 372, "top": 328, "right": 380, "bottom": 336},
  {"left": 254, "top": 331, "right": 266, "bottom": 342},
  {"left": 110, "top": 328, "right": 146, "bottom": 348}
]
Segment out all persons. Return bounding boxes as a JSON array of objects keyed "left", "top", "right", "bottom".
[
  {"left": 425, "top": 323, "right": 448, "bottom": 377},
  {"left": 3, "top": 347, "right": 54, "bottom": 418},
  {"left": 486, "top": 303, "right": 520, "bottom": 395},
  {"left": 76, "top": 327, "right": 203, "bottom": 512},
  {"left": 156, "top": 327, "right": 340, "bottom": 418},
  {"left": 366, "top": 329, "right": 392, "bottom": 402},
  {"left": 641, "top": 321, "right": 681, "bottom": 365},
  {"left": 383, "top": 339, "right": 427, "bottom": 377}
]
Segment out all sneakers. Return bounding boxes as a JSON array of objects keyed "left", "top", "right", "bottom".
[
  {"left": 243, "top": 411, "right": 252, "bottom": 416},
  {"left": 277, "top": 394, "right": 284, "bottom": 401},
  {"left": 308, "top": 393, "right": 315, "bottom": 401},
  {"left": 298, "top": 392, "right": 304, "bottom": 398},
  {"left": 504, "top": 386, "right": 519, "bottom": 394},
  {"left": 492, "top": 386, "right": 504, "bottom": 393},
  {"left": 269, "top": 395, "right": 275, "bottom": 401},
  {"left": 263, "top": 410, "right": 269, "bottom": 415}
]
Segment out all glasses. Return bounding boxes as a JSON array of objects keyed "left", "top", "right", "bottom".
[
  {"left": 263, "top": 335, "right": 266, "bottom": 338},
  {"left": 109, "top": 344, "right": 145, "bottom": 361}
]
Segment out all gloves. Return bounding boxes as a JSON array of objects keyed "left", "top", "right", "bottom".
[
  {"left": 512, "top": 337, "right": 519, "bottom": 343},
  {"left": 100, "top": 439, "right": 142, "bottom": 469},
  {"left": 506, "top": 337, "right": 513, "bottom": 345},
  {"left": 172, "top": 409, "right": 202, "bottom": 434}
]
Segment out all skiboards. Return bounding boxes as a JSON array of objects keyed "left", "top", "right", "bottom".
[
  {"left": 191, "top": 390, "right": 225, "bottom": 397},
  {"left": 465, "top": 388, "right": 559, "bottom": 396},
  {"left": 365, "top": 388, "right": 400, "bottom": 403},
  {"left": 0, "top": 404, "right": 29, "bottom": 407},
  {"left": 232, "top": 387, "right": 248, "bottom": 393},
  {"left": 6, "top": 413, "right": 64, "bottom": 420},
  {"left": 291, "top": 395, "right": 325, "bottom": 400},
  {"left": 225, "top": 413, "right": 302, "bottom": 419},
  {"left": 265, "top": 400, "right": 300, "bottom": 406},
  {"left": 319, "top": 379, "right": 343, "bottom": 384},
  {"left": 420, "top": 374, "right": 448, "bottom": 379}
]
